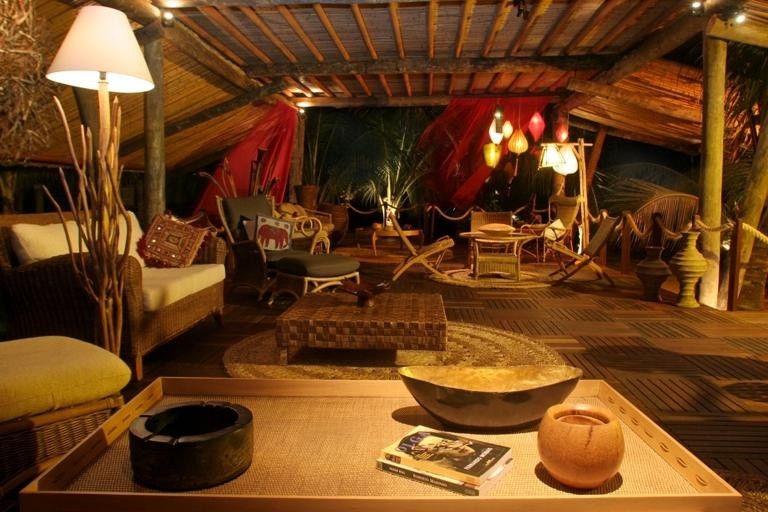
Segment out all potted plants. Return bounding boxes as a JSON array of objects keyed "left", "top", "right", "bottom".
[
  {"left": 319, "top": 163, "right": 353, "bottom": 248},
  {"left": 299, "top": 110, "right": 323, "bottom": 209},
  {"left": 355, "top": 122, "right": 432, "bottom": 230}
]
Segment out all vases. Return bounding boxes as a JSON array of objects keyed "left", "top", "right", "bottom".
[
  {"left": 634, "top": 246, "right": 671, "bottom": 300},
  {"left": 669, "top": 230, "right": 708, "bottom": 309}
]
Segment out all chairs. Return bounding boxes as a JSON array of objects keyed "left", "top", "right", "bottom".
[
  {"left": 468, "top": 210, "right": 513, "bottom": 270},
  {"left": 214, "top": 194, "right": 322, "bottom": 306},
  {"left": 274, "top": 202, "right": 335, "bottom": 254},
  {"left": 546, "top": 216, "right": 619, "bottom": 287},
  {"left": 520, "top": 194, "right": 581, "bottom": 262},
  {"left": 389, "top": 213, "right": 454, "bottom": 282}
]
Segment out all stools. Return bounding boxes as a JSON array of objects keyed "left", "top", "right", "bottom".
[
  {"left": 0, "top": 393, "right": 126, "bottom": 503},
  {"left": 267, "top": 253, "right": 360, "bottom": 307}
]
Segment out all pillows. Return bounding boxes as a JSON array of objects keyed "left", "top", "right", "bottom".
[
  {"left": 9, "top": 219, "right": 89, "bottom": 265},
  {"left": 0, "top": 334, "right": 132, "bottom": 424},
  {"left": 83, "top": 210, "right": 147, "bottom": 268}
]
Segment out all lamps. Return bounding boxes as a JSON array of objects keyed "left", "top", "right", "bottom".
[
  {"left": 528, "top": 98, "right": 546, "bottom": 142},
  {"left": 538, "top": 138, "right": 593, "bottom": 248},
  {"left": 507, "top": 98, "right": 528, "bottom": 157},
  {"left": 488, "top": 118, "right": 503, "bottom": 145},
  {"left": 493, "top": 100, "right": 503, "bottom": 121},
  {"left": 555, "top": 109, "right": 570, "bottom": 142},
  {"left": 483, "top": 143, "right": 502, "bottom": 168},
  {"left": 502, "top": 99, "right": 514, "bottom": 139},
  {"left": 43, "top": 6, "right": 156, "bottom": 248}
]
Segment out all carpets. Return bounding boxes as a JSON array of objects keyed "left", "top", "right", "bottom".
[
  {"left": 429, "top": 268, "right": 553, "bottom": 289},
  {"left": 221, "top": 321, "right": 565, "bottom": 379}
]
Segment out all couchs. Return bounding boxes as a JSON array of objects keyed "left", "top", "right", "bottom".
[{"left": 1, "top": 205, "right": 227, "bottom": 382}]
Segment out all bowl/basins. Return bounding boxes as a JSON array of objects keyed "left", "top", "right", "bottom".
[{"left": 396, "top": 363, "right": 583, "bottom": 429}]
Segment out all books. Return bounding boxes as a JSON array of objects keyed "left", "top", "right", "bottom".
[{"left": 376, "top": 424, "right": 514, "bottom": 496}]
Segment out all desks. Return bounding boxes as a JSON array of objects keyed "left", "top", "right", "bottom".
[
  {"left": 355, "top": 228, "right": 425, "bottom": 257},
  {"left": 459, "top": 231, "right": 537, "bottom": 282}
]
